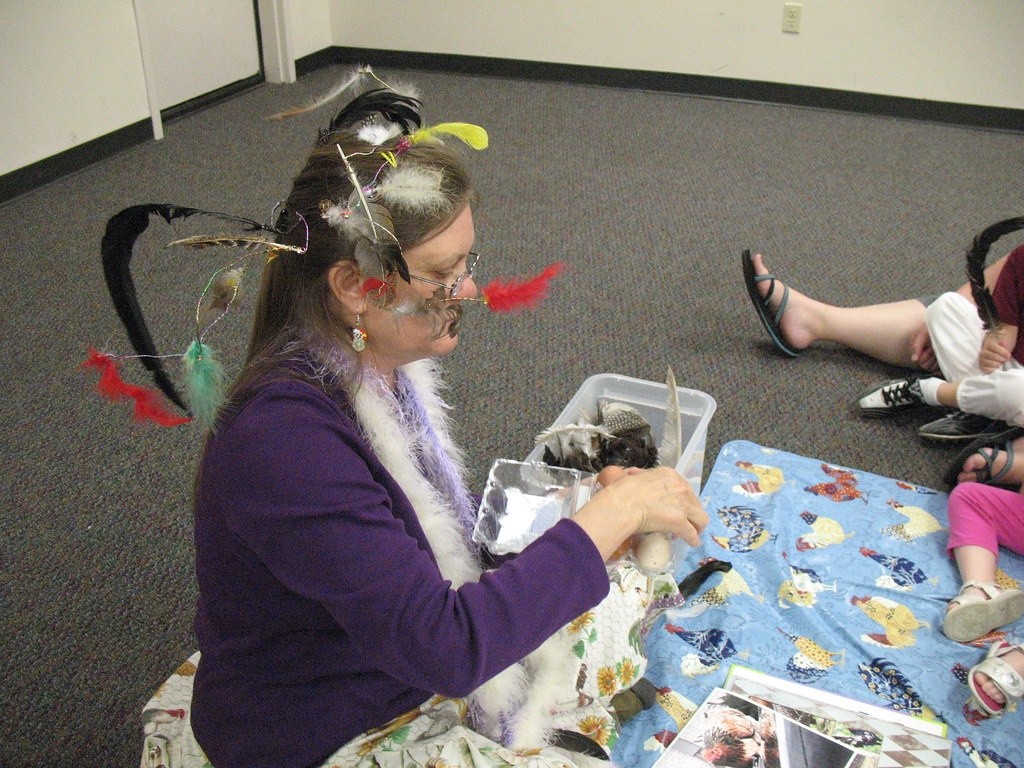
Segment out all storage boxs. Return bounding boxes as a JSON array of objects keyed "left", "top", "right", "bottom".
[{"left": 471, "top": 371, "right": 718, "bottom": 569}]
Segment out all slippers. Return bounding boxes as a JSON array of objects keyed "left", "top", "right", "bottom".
[
  {"left": 944, "top": 428, "right": 1022, "bottom": 486},
  {"left": 739, "top": 248, "right": 801, "bottom": 358}
]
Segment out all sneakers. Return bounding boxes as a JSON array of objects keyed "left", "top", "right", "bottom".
[
  {"left": 850, "top": 371, "right": 943, "bottom": 417},
  {"left": 918, "top": 411, "right": 1006, "bottom": 445}
]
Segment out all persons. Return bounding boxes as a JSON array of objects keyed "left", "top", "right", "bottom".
[
  {"left": 742, "top": 244, "right": 1024, "bottom": 716},
  {"left": 191, "top": 134, "right": 709, "bottom": 768}
]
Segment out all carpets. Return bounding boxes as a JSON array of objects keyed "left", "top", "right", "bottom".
[{"left": 609, "top": 438, "right": 1024, "bottom": 768}]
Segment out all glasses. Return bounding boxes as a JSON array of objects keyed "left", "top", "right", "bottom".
[{"left": 401, "top": 249, "right": 481, "bottom": 301}]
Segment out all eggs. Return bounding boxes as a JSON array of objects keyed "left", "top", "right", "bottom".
[
  {"left": 595, "top": 465, "right": 624, "bottom": 487},
  {"left": 638, "top": 531, "right": 671, "bottom": 570}
]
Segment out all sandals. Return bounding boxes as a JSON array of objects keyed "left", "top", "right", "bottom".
[
  {"left": 967, "top": 640, "right": 1024, "bottom": 716},
  {"left": 942, "top": 580, "right": 1024, "bottom": 643}
]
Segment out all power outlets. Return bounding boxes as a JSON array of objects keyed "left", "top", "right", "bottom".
[{"left": 782, "top": 3, "right": 803, "bottom": 33}]
